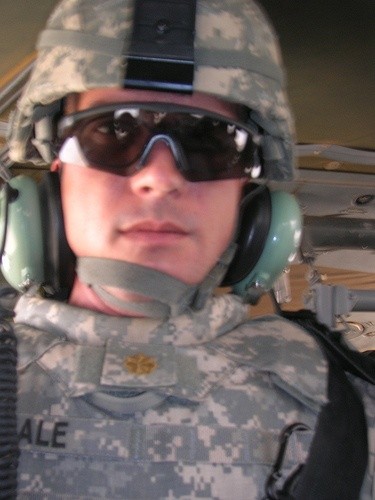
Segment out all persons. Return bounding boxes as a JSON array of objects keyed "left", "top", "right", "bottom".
[{"left": 0, "top": 0, "right": 374, "bottom": 500}]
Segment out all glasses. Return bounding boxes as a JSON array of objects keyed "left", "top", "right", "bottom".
[{"left": 52, "top": 102, "right": 261, "bottom": 183}]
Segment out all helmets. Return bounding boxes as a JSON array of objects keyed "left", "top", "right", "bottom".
[{"left": 7, "top": 0, "right": 300, "bottom": 193}]
